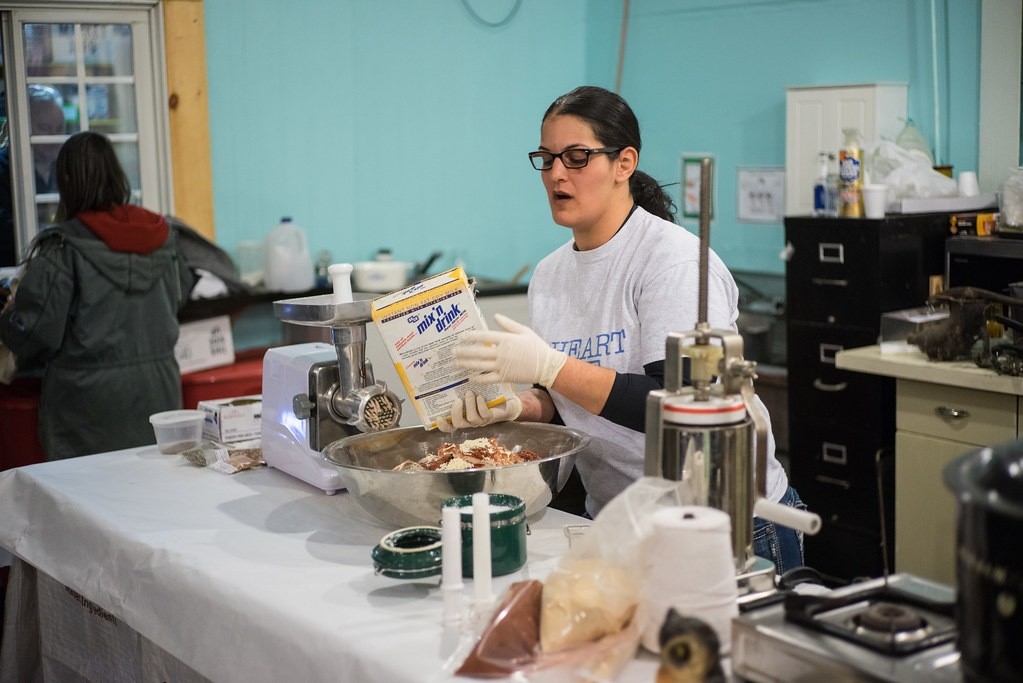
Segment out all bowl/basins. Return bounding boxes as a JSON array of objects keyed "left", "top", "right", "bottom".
[
  {"left": 149, "top": 409, "right": 206, "bottom": 455},
  {"left": 351, "top": 261, "right": 416, "bottom": 294},
  {"left": 319, "top": 420, "right": 591, "bottom": 528}
]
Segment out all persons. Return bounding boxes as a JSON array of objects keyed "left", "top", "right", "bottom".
[
  {"left": 435, "top": 86, "right": 808, "bottom": 576},
  {"left": 1, "top": 85, "right": 183, "bottom": 461}
]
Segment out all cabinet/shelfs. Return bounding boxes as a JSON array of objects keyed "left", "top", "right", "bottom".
[
  {"left": 783, "top": 81, "right": 912, "bottom": 215},
  {"left": 783, "top": 207, "right": 1000, "bottom": 565}
]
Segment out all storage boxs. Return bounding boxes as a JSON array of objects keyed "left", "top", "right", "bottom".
[
  {"left": 371, "top": 267, "right": 518, "bottom": 431},
  {"left": 197, "top": 394, "right": 266, "bottom": 439},
  {"left": 174, "top": 316, "right": 234, "bottom": 376}
]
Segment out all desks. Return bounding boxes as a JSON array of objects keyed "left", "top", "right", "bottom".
[
  {"left": 834, "top": 338, "right": 1023, "bottom": 588},
  {"left": 0, "top": 433, "right": 828, "bottom": 683}
]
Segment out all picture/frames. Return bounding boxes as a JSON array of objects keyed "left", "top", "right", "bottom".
[{"left": 682, "top": 152, "right": 718, "bottom": 220}]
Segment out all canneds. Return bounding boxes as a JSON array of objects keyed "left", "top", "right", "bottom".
[{"left": 838, "top": 147, "right": 865, "bottom": 218}]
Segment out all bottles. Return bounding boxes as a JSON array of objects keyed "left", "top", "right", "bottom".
[
  {"left": 376, "top": 249, "right": 392, "bottom": 262},
  {"left": 813, "top": 151, "right": 839, "bottom": 218},
  {"left": 237, "top": 218, "right": 333, "bottom": 295}
]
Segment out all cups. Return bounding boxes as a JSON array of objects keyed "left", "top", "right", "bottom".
[
  {"left": 958, "top": 171, "right": 981, "bottom": 197},
  {"left": 440, "top": 493, "right": 528, "bottom": 577},
  {"left": 858, "top": 184, "right": 888, "bottom": 219}
]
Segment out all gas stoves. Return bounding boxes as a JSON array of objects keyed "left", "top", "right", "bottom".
[{"left": 729, "top": 572, "right": 964, "bottom": 683}]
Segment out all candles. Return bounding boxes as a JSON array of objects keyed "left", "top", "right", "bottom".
[
  {"left": 471, "top": 491, "right": 492, "bottom": 599},
  {"left": 441, "top": 504, "right": 462, "bottom": 587}
]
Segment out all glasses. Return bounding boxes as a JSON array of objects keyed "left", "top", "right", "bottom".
[{"left": 528, "top": 147, "right": 620, "bottom": 170}]
